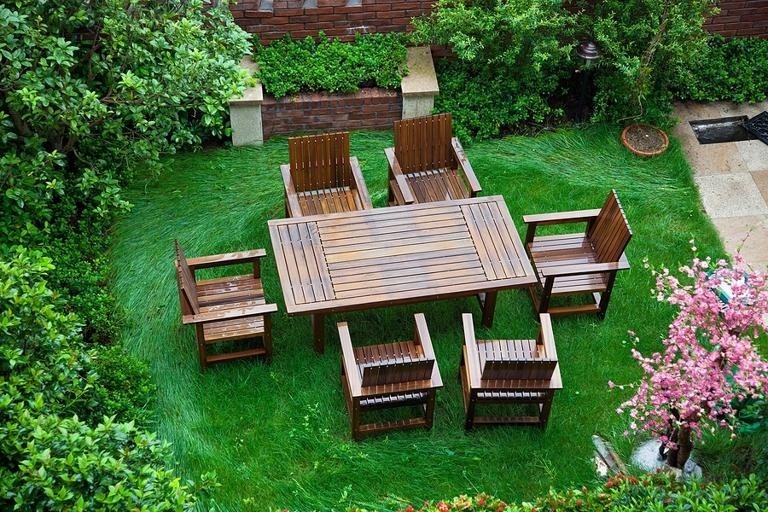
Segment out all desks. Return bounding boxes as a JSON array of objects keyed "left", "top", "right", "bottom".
[{"left": 267, "top": 195, "right": 541, "bottom": 354}]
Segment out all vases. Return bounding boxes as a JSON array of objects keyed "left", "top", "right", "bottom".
[{"left": 622, "top": 123, "right": 669, "bottom": 158}]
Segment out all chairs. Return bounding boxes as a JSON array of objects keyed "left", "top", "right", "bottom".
[
  {"left": 334, "top": 312, "right": 563, "bottom": 441},
  {"left": 169, "top": 238, "right": 279, "bottom": 372},
  {"left": 522, "top": 188, "right": 635, "bottom": 322},
  {"left": 279, "top": 112, "right": 482, "bottom": 217}
]
